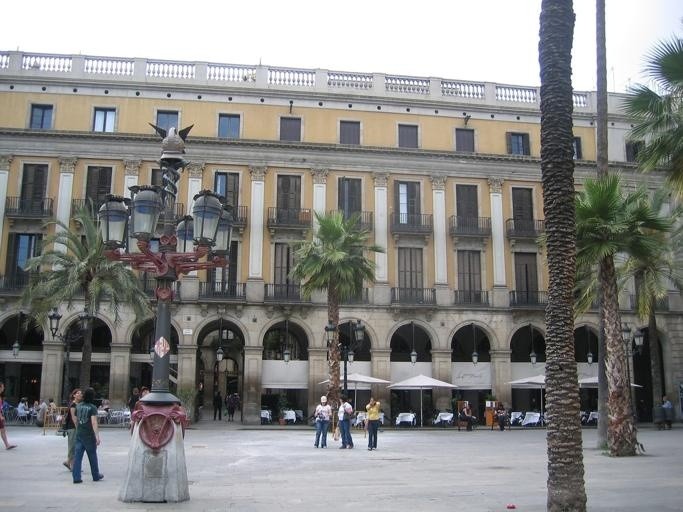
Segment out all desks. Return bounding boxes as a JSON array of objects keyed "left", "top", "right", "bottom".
[{"left": 260, "top": 410, "right": 600, "bottom": 427}]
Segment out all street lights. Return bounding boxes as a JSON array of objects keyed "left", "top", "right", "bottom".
[
  {"left": 48, "top": 308, "right": 69, "bottom": 402},
  {"left": 324, "top": 320, "right": 364, "bottom": 402},
  {"left": 621, "top": 322, "right": 643, "bottom": 418},
  {"left": 95, "top": 124, "right": 233, "bottom": 502}
]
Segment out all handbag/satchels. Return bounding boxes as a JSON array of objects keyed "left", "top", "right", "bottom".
[
  {"left": 343, "top": 413, "right": 349, "bottom": 422},
  {"left": 333, "top": 424, "right": 340, "bottom": 441}
]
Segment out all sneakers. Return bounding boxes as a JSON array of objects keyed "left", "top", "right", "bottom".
[
  {"left": 313, "top": 446, "right": 317, "bottom": 449},
  {"left": 367, "top": 448, "right": 371, "bottom": 450},
  {"left": 322, "top": 446, "right": 326, "bottom": 449},
  {"left": 338, "top": 445, "right": 345, "bottom": 449},
  {"left": 346, "top": 445, "right": 351, "bottom": 449},
  {"left": 371, "top": 447, "right": 375, "bottom": 450}
]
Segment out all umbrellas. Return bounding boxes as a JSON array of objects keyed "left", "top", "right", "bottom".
[
  {"left": 579, "top": 377, "right": 645, "bottom": 389},
  {"left": 387, "top": 374, "right": 458, "bottom": 428},
  {"left": 317, "top": 373, "right": 391, "bottom": 421},
  {"left": 507, "top": 374, "right": 546, "bottom": 427}
]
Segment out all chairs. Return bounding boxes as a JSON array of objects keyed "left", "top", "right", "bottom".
[{"left": 0, "top": 398, "right": 133, "bottom": 426}]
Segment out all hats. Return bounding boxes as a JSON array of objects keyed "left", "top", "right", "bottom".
[{"left": 320, "top": 396, "right": 327, "bottom": 403}]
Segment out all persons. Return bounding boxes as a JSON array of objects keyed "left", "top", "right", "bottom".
[
  {"left": 62, "top": 390, "right": 83, "bottom": 471},
  {"left": 0, "top": 381, "right": 150, "bottom": 450},
  {"left": 494, "top": 404, "right": 507, "bottom": 430},
  {"left": 338, "top": 395, "right": 353, "bottom": 450},
  {"left": 314, "top": 395, "right": 332, "bottom": 449},
  {"left": 661, "top": 395, "right": 672, "bottom": 428},
  {"left": 213, "top": 391, "right": 240, "bottom": 422},
  {"left": 460, "top": 403, "right": 477, "bottom": 432},
  {"left": 71, "top": 388, "right": 104, "bottom": 484},
  {"left": 365, "top": 397, "right": 381, "bottom": 450}
]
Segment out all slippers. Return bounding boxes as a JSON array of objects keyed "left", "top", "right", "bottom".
[{"left": 63, "top": 462, "right": 71, "bottom": 470}]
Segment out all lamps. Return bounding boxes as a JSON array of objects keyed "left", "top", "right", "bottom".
[
  {"left": 347, "top": 320, "right": 355, "bottom": 362},
  {"left": 471, "top": 325, "right": 479, "bottom": 364},
  {"left": 409, "top": 322, "right": 418, "bottom": 363},
  {"left": 530, "top": 327, "right": 537, "bottom": 364},
  {"left": 587, "top": 328, "right": 593, "bottom": 363},
  {"left": 283, "top": 320, "right": 290, "bottom": 362},
  {"left": 217, "top": 318, "right": 223, "bottom": 361},
  {"left": 150, "top": 318, "right": 155, "bottom": 359},
  {"left": 12, "top": 313, "right": 21, "bottom": 356}
]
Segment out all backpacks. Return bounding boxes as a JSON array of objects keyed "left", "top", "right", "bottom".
[{"left": 61, "top": 403, "right": 75, "bottom": 437}]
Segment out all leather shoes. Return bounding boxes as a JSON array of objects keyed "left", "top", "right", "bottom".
[
  {"left": 74, "top": 480, "right": 82, "bottom": 483},
  {"left": 93, "top": 474, "right": 103, "bottom": 481}
]
